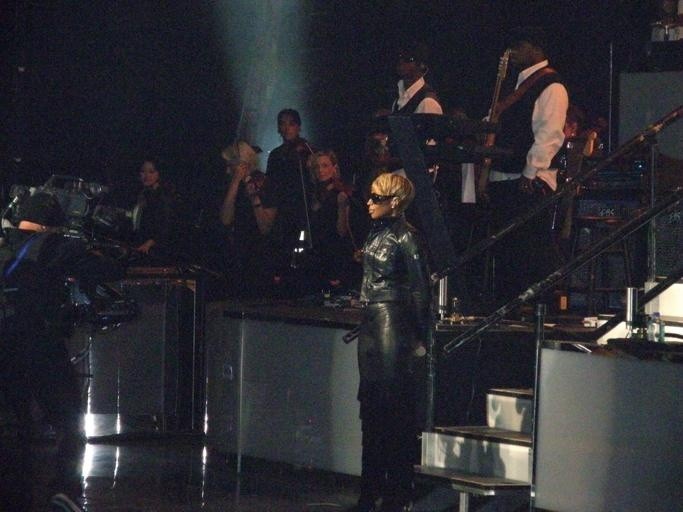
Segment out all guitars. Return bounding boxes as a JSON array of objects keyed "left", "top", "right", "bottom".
[{"left": 478, "top": 49, "right": 510, "bottom": 193}]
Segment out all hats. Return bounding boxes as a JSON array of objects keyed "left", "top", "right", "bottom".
[{"left": 17, "top": 192, "right": 66, "bottom": 228}]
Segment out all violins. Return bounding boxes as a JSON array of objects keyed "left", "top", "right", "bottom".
[{"left": 317, "top": 177, "right": 362, "bottom": 205}]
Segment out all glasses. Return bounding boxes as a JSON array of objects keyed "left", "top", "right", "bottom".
[{"left": 368, "top": 192, "right": 396, "bottom": 202}]
